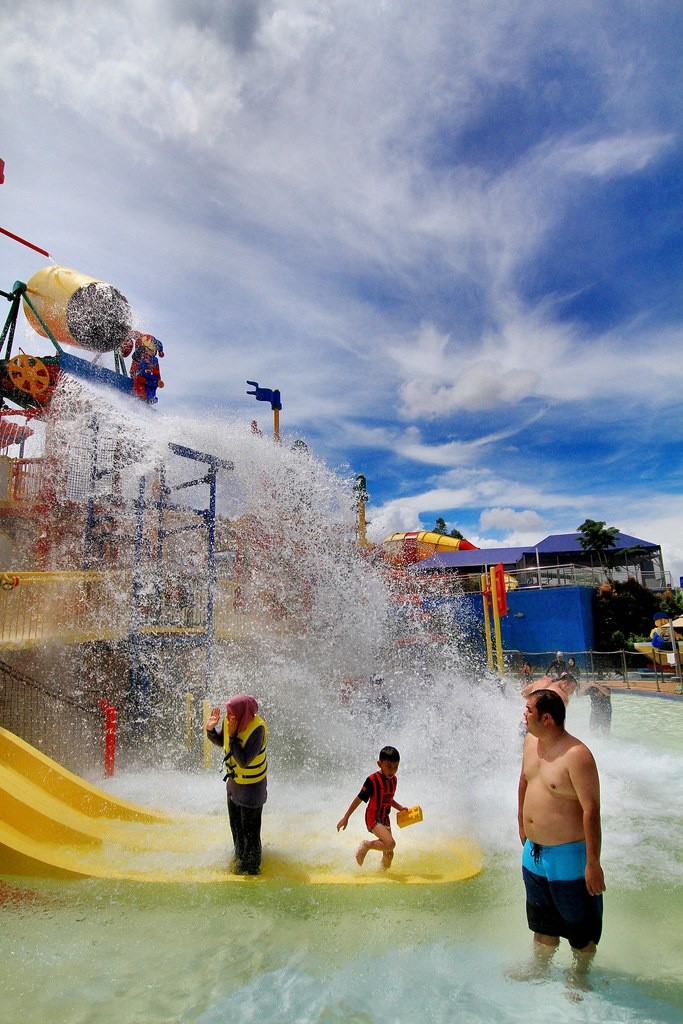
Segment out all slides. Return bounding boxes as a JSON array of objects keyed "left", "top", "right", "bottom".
[{"left": 0, "top": 725, "right": 486, "bottom": 886}]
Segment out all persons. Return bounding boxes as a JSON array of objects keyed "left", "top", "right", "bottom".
[
  {"left": 545, "top": 653, "right": 582, "bottom": 698},
  {"left": 206, "top": 694, "right": 268, "bottom": 874},
  {"left": 647, "top": 612, "right": 683, "bottom": 673},
  {"left": 522, "top": 674, "right": 578, "bottom": 707},
  {"left": 579, "top": 681, "right": 612, "bottom": 734},
  {"left": 337, "top": 746, "right": 410, "bottom": 868},
  {"left": 517, "top": 689, "right": 606, "bottom": 951},
  {"left": 520, "top": 662, "right": 533, "bottom": 689}
]
